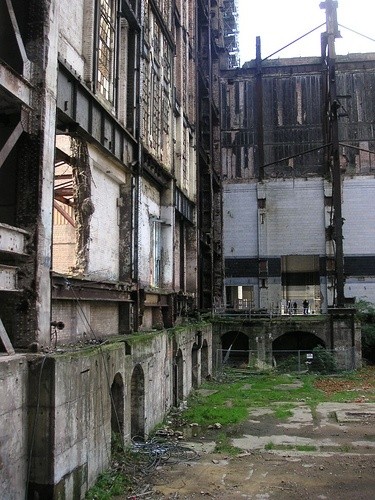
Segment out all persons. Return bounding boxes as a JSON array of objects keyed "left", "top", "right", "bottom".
[
  {"left": 287, "top": 300, "right": 291, "bottom": 313},
  {"left": 292, "top": 301, "right": 297, "bottom": 314},
  {"left": 303, "top": 299, "right": 309, "bottom": 314}
]
